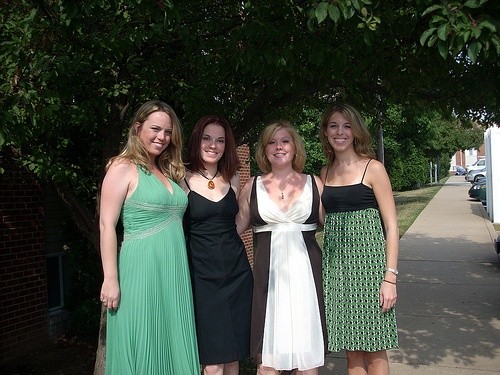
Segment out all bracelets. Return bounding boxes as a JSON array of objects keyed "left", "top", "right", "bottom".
[
  {"left": 385, "top": 267, "right": 399, "bottom": 275},
  {"left": 383, "top": 280, "right": 397, "bottom": 285}
]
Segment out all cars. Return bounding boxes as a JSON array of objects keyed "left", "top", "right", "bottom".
[
  {"left": 455, "top": 166, "right": 465, "bottom": 175},
  {"left": 468, "top": 176, "right": 486, "bottom": 201},
  {"left": 465, "top": 166, "right": 486, "bottom": 185}
]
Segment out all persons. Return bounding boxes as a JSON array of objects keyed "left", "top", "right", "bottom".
[
  {"left": 235, "top": 120, "right": 331, "bottom": 375},
  {"left": 96, "top": 101, "right": 201, "bottom": 375},
  {"left": 319, "top": 103, "right": 399, "bottom": 375},
  {"left": 178, "top": 116, "right": 253, "bottom": 375}
]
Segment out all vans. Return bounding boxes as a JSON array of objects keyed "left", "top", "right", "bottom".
[{"left": 465, "top": 159, "right": 485, "bottom": 175}]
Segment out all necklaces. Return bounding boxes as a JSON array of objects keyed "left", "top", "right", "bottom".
[
  {"left": 269, "top": 171, "right": 296, "bottom": 199},
  {"left": 197, "top": 168, "right": 219, "bottom": 189}
]
